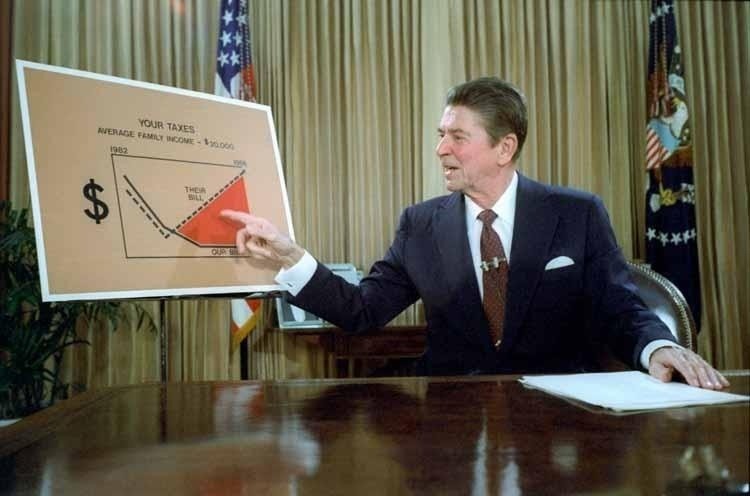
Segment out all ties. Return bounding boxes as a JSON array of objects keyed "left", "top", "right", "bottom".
[{"left": 476, "top": 209, "right": 509, "bottom": 357}]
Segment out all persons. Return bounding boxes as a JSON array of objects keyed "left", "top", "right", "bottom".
[{"left": 217, "top": 77, "right": 731, "bottom": 391}]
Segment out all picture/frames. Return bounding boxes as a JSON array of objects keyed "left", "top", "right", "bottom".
[{"left": 276, "top": 264, "right": 358, "bottom": 329}]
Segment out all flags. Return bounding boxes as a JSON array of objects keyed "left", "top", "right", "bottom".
[
  {"left": 643, "top": 2, "right": 703, "bottom": 336},
  {"left": 218, "top": 0, "right": 260, "bottom": 353}
]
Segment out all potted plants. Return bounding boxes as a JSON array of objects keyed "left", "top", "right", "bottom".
[{"left": 1, "top": 203, "right": 158, "bottom": 428}]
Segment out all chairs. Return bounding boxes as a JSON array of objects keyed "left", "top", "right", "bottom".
[{"left": 626, "top": 260, "right": 697, "bottom": 351}]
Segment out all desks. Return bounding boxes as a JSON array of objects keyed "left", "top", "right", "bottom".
[
  {"left": 0, "top": 375, "right": 748, "bottom": 494},
  {"left": 298, "top": 327, "right": 426, "bottom": 378}
]
